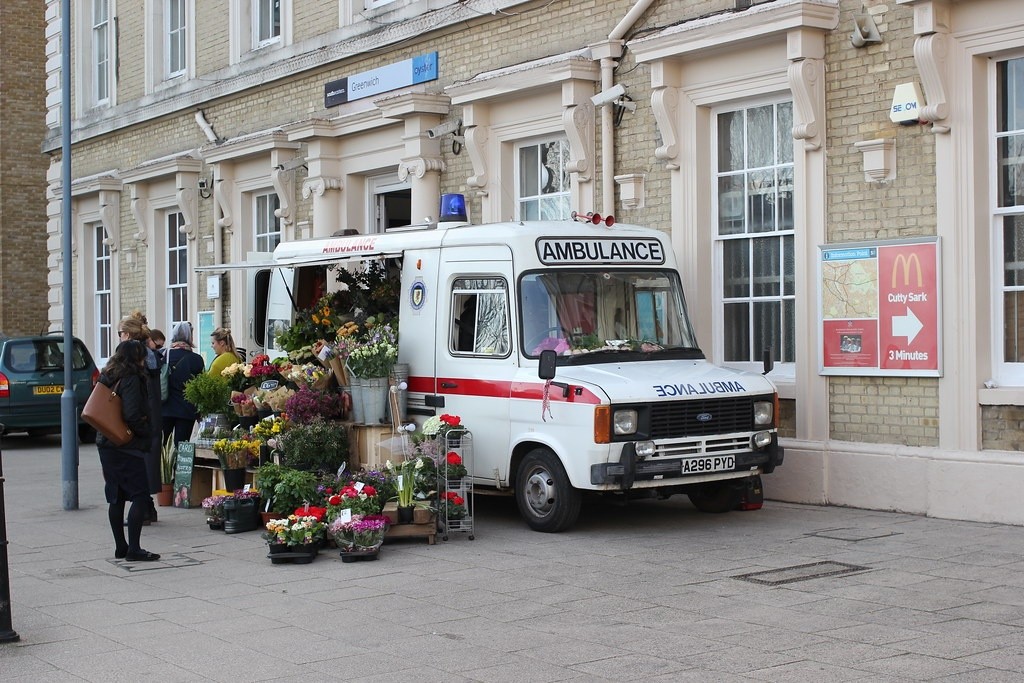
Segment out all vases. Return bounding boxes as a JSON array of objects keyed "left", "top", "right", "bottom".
[
  {"left": 269, "top": 543, "right": 318, "bottom": 564},
  {"left": 343, "top": 556, "right": 357, "bottom": 562},
  {"left": 361, "top": 555, "right": 377, "bottom": 561},
  {"left": 286, "top": 463, "right": 305, "bottom": 471},
  {"left": 257, "top": 410, "right": 271, "bottom": 422},
  {"left": 271, "top": 411, "right": 282, "bottom": 417},
  {"left": 224, "top": 469, "right": 246, "bottom": 492},
  {"left": 399, "top": 506, "right": 415, "bottom": 522},
  {"left": 239, "top": 416, "right": 258, "bottom": 430}
]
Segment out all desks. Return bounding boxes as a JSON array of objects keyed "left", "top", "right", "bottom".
[{"left": 336, "top": 418, "right": 414, "bottom": 468}]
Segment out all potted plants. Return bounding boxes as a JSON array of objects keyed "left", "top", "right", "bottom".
[
  {"left": 182, "top": 367, "right": 233, "bottom": 440},
  {"left": 155, "top": 426, "right": 177, "bottom": 505},
  {"left": 254, "top": 461, "right": 317, "bottom": 530}
]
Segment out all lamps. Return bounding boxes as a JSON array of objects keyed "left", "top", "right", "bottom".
[
  {"left": 297, "top": 221, "right": 313, "bottom": 239},
  {"left": 202, "top": 235, "right": 215, "bottom": 253},
  {"left": 854, "top": 138, "right": 894, "bottom": 183},
  {"left": 122, "top": 247, "right": 137, "bottom": 272},
  {"left": 614, "top": 174, "right": 644, "bottom": 209}
]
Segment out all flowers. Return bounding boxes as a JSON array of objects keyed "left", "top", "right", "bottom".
[
  {"left": 531, "top": 336, "right": 664, "bottom": 356},
  {"left": 202, "top": 261, "right": 469, "bottom": 553}
]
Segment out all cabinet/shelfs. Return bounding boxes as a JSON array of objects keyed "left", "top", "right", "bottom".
[{"left": 436, "top": 429, "right": 475, "bottom": 541}]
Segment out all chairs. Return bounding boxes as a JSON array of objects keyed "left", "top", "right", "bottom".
[
  {"left": 556, "top": 293, "right": 595, "bottom": 338},
  {"left": 49, "top": 353, "right": 64, "bottom": 366},
  {"left": 459, "top": 295, "right": 476, "bottom": 351}
]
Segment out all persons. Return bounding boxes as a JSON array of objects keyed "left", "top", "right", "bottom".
[
  {"left": 94, "top": 337, "right": 161, "bottom": 562},
  {"left": 160, "top": 320, "right": 205, "bottom": 453},
  {"left": 118, "top": 312, "right": 164, "bottom": 527},
  {"left": 145, "top": 328, "right": 167, "bottom": 352},
  {"left": 206, "top": 326, "right": 243, "bottom": 383}
]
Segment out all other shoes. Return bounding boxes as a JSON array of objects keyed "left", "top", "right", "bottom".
[
  {"left": 125, "top": 548, "right": 160, "bottom": 561},
  {"left": 124, "top": 517, "right": 151, "bottom": 526},
  {"left": 115, "top": 549, "right": 128, "bottom": 559},
  {"left": 150, "top": 517, "right": 157, "bottom": 523}
]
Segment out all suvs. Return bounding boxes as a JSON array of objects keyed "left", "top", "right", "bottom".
[{"left": 0, "top": 330, "right": 102, "bottom": 446}]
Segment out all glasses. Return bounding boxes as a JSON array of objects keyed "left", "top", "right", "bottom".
[
  {"left": 151, "top": 339, "right": 163, "bottom": 349},
  {"left": 118, "top": 331, "right": 125, "bottom": 337}
]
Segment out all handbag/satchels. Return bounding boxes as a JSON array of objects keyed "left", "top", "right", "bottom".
[
  {"left": 81, "top": 379, "right": 133, "bottom": 445},
  {"left": 159, "top": 348, "right": 171, "bottom": 403}
]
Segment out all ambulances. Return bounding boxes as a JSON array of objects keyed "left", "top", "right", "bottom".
[{"left": 193, "top": 192, "right": 784, "bottom": 535}]
